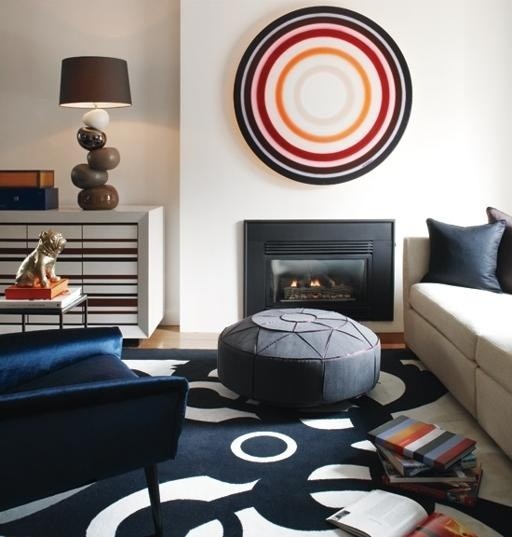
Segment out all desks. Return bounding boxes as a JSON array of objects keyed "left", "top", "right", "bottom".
[{"left": 0, "top": 295, "right": 89, "bottom": 332}]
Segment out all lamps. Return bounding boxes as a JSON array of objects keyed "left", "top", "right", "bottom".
[{"left": 57, "top": 54, "right": 133, "bottom": 210}]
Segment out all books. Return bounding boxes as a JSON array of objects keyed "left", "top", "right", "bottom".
[
  {"left": 0, "top": 288, "right": 81, "bottom": 309},
  {"left": 325, "top": 414, "right": 504, "bottom": 537}
]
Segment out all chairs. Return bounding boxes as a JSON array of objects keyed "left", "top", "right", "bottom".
[{"left": 0, "top": 326, "right": 192, "bottom": 536}]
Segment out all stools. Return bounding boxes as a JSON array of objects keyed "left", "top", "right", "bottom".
[{"left": 216, "top": 306, "right": 385, "bottom": 408}]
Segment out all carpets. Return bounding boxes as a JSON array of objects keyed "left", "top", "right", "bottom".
[{"left": 0, "top": 340, "right": 512, "bottom": 537}]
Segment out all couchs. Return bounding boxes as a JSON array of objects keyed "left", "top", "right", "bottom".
[{"left": 402, "top": 235, "right": 512, "bottom": 462}]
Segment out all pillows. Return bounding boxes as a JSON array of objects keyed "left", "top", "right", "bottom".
[
  {"left": 419, "top": 216, "right": 507, "bottom": 294},
  {"left": 486, "top": 205, "right": 512, "bottom": 294}
]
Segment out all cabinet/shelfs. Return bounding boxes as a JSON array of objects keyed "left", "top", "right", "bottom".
[{"left": 0, "top": 206, "right": 167, "bottom": 347}]
